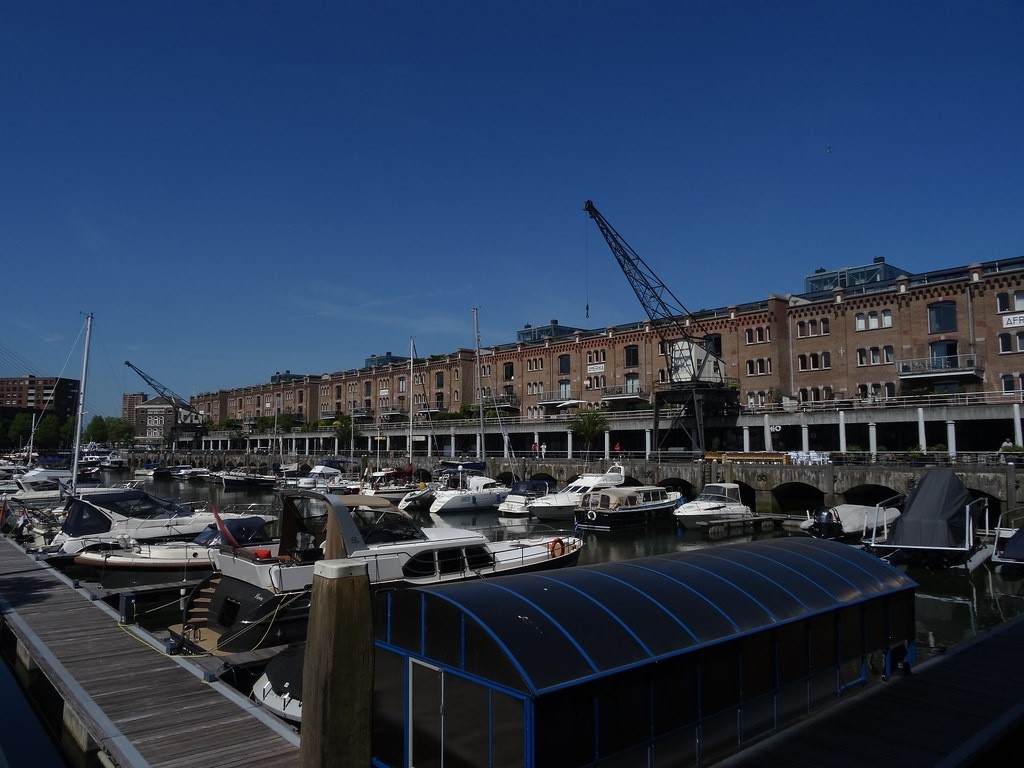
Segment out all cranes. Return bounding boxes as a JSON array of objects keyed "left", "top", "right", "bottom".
[
  {"left": 123, "top": 360, "right": 209, "bottom": 452},
  {"left": 581, "top": 197, "right": 743, "bottom": 460}
]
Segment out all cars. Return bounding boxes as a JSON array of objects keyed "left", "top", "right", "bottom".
[{"left": 991, "top": 506, "right": 1024, "bottom": 564}]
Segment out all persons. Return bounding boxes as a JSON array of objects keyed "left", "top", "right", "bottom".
[
  {"left": 612, "top": 442, "right": 620, "bottom": 460},
  {"left": 532, "top": 442, "right": 546, "bottom": 461}
]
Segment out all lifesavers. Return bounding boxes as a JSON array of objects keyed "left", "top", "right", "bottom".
[
  {"left": 550, "top": 538, "right": 566, "bottom": 558},
  {"left": 587, "top": 510, "right": 597, "bottom": 521}
]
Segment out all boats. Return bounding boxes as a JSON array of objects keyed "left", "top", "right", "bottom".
[
  {"left": 526, "top": 462, "right": 627, "bottom": 519},
  {"left": 497, "top": 481, "right": 560, "bottom": 515},
  {"left": 798, "top": 502, "right": 901, "bottom": 540},
  {"left": 73, "top": 515, "right": 270, "bottom": 590},
  {"left": 345, "top": 532, "right": 923, "bottom": 768},
  {"left": 205, "top": 484, "right": 586, "bottom": 644},
  {"left": 672, "top": 482, "right": 754, "bottom": 530},
  {"left": 573, "top": 484, "right": 685, "bottom": 530},
  {"left": 424, "top": 460, "right": 513, "bottom": 514},
  {"left": 870, "top": 467, "right": 990, "bottom": 553},
  {"left": 0, "top": 312, "right": 435, "bottom": 567}
]
